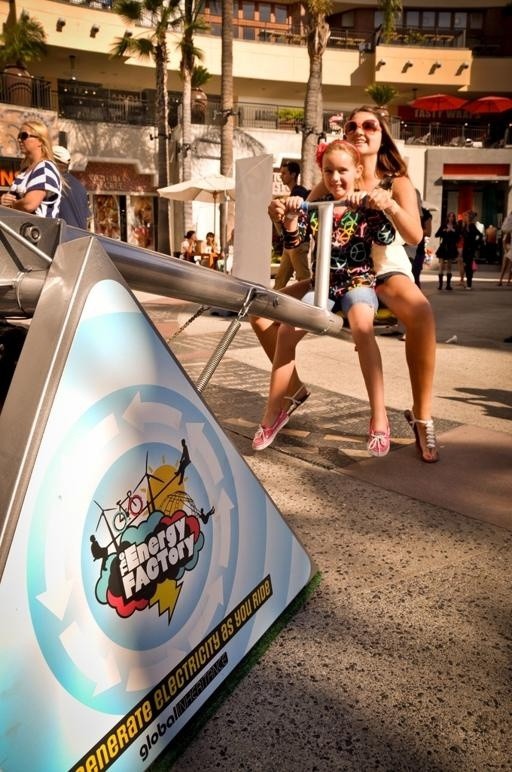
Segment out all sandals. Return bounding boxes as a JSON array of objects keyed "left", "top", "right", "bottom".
[
  {"left": 284, "top": 384, "right": 311, "bottom": 416},
  {"left": 403, "top": 410, "right": 440, "bottom": 462}
]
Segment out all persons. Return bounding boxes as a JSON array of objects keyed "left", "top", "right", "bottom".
[
  {"left": 0, "top": 120, "right": 65, "bottom": 221},
  {"left": 248, "top": 105, "right": 442, "bottom": 463},
  {"left": 275, "top": 160, "right": 310, "bottom": 290},
  {"left": 400, "top": 182, "right": 432, "bottom": 292},
  {"left": 327, "top": 109, "right": 344, "bottom": 133},
  {"left": 247, "top": 139, "right": 399, "bottom": 460},
  {"left": 217, "top": 229, "right": 234, "bottom": 271},
  {"left": 49, "top": 144, "right": 94, "bottom": 234},
  {"left": 200, "top": 232, "right": 220, "bottom": 269},
  {"left": 434, "top": 206, "right": 512, "bottom": 293},
  {"left": 178, "top": 230, "right": 197, "bottom": 262}
]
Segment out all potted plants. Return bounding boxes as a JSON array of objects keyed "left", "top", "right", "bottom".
[
  {"left": 0, "top": 4, "right": 53, "bottom": 109},
  {"left": 174, "top": 62, "right": 214, "bottom": 126}
]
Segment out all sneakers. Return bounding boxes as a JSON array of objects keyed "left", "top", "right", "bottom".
[
  {"left": 250, "top": 409, "right": 290, "bottom": 451},
  {"left": 497, "top": 280, "right": 512, "bottom": 287},
  {"left": 368, "top": 414, "right": 392, "bottom": 456},
  {"left": 455, "top": 282, "right": 473, "bottom": 291}
]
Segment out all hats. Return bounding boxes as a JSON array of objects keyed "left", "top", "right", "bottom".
[{"left": 49, "top": 145, "right": 73, "bottom": 165}]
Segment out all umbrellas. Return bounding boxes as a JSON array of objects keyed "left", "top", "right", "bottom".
[
  {"left": 460, "top": 96, "right": 512, "bottom": 147},
  {"left": 157, "top": 172, "right": 235, "bottom": 262},
  {"left": 404, "top": 94, "right": 466, "bottom": 146}
]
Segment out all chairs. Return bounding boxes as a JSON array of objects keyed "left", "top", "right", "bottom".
[
  {"left": 403, "top": 132, "right": 485, "bottom": 149},
  {"left": 170, "top": 243, "right": 224, "bottom": 272}
]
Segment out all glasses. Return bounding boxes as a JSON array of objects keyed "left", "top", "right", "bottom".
[
  {"left": 17, "top": 132, "right": 40, "bottom": 141},
  {"left": 343, "top": 119, "right": 380, "bottom": 135}
]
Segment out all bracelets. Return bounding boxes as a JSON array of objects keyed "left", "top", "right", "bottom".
[{"left": 384, "top": 199, "right": 400, "bottom": 218}]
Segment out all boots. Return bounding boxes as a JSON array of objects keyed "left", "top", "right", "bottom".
[
  {"left": 446, "top": 272, "right": 454, "bottom": 291},
  {"left": 437, "top": 273, "right": 444, "bottom": 290}
]
